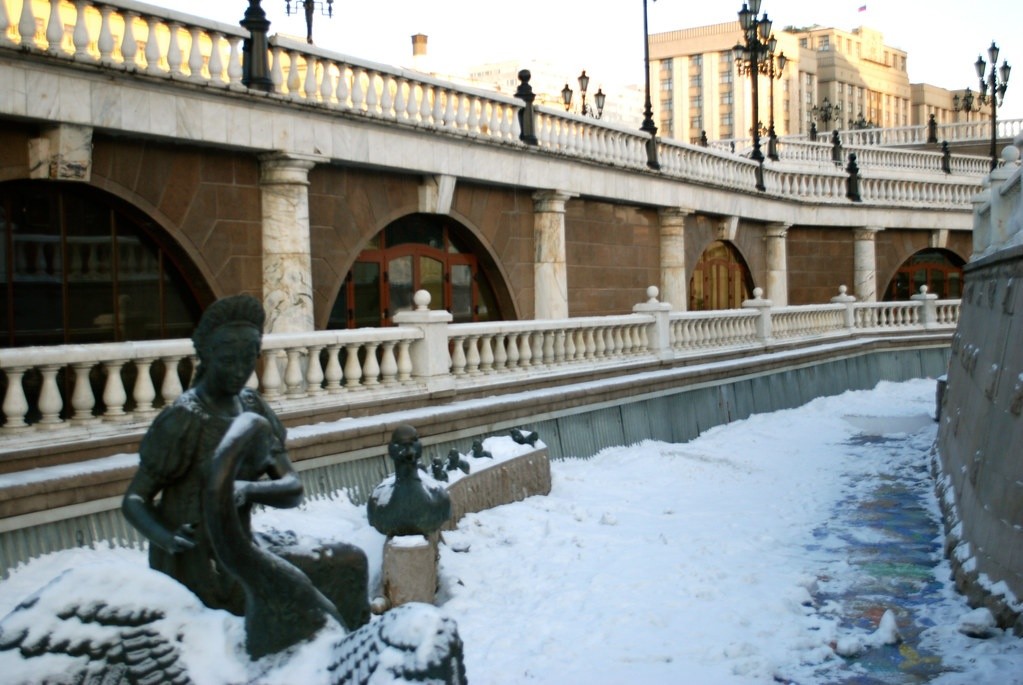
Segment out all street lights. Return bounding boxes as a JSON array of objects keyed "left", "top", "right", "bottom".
[
  {"left": 812, "top": 96, "right": 841, "bottom": 132},
  {"left": 952, "top": 88, "right": 983, "bottom": 136},
  {"left": 974, "top": 42, "right": 1012, "bottom": 156},
  {"left": 733, "top": 0, "right": 787, "bottom": 167}
]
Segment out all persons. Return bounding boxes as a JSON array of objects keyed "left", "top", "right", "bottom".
[{"left": 122, "top": 292, "right": 371, "bottom": 658}]
